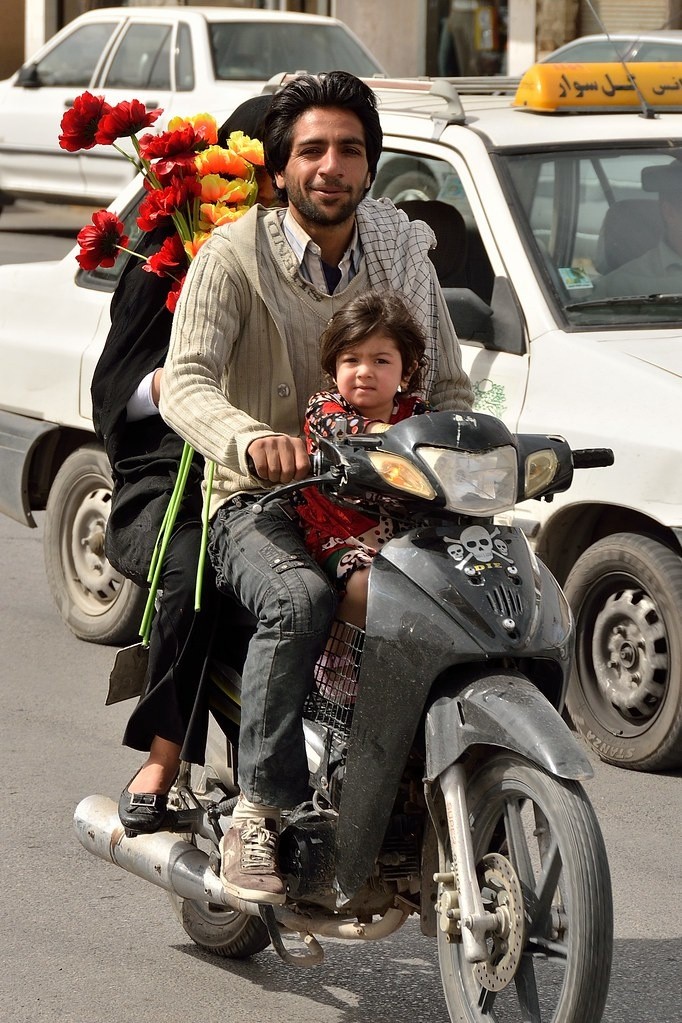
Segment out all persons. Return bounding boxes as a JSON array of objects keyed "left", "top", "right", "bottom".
[
  {"left": 591, "top": 159, "right": 682, "bottom": 300},
  {"left": 158, "top": 71, "right": 474, "bottom": 905},
  {"left": 294, "top": 290, "right": 441, "bottom": 709},
  {"left": 89, "top": 96, "right": 290, "bottom": 830}
]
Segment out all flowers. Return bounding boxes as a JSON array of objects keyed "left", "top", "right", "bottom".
[{"left": 58, "top": 92, "right": 264, "bottom": 647}]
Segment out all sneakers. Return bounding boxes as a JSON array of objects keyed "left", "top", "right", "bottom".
[{"left": 219, "top": 818, "right": 286, "bottom": 905}]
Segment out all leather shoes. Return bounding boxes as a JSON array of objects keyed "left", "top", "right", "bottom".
[{"left": 118, "top": 763, "right": 180, "bottom": 832}]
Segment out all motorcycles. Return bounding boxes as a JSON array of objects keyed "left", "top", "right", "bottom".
[{"left": 70, "top": 403, "right": 614, "bottom": 1023}]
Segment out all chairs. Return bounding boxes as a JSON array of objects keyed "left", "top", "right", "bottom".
[
  {"left": 396, "top": 198, "right": 490, "bottom": 307},
  {"left": 590, "top": 197, "right": 659, "bottom": 274}
]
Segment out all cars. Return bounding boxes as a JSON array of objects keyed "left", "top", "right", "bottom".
[
  {"left": 0, "top": 6, "right": 391, "bottom": 213},
  {"left": 520, "top": 29, "right": 682, "bottom": 189},
  {"left": 1, "top": 61, "right": 682, "bottom": 772}
]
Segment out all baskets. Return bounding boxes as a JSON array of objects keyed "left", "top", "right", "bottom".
[{"left": 302, "top": 616, "right": 367, "bottom": 736}]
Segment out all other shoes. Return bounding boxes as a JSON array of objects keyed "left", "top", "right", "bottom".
[{"left": 314, "top": 653, "right": 357, "bottom": 706}]
